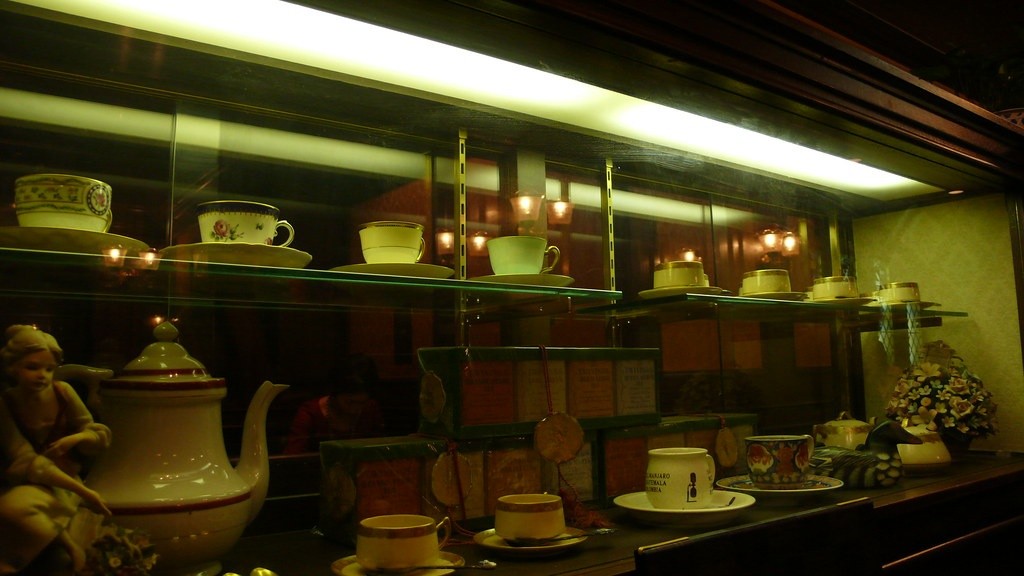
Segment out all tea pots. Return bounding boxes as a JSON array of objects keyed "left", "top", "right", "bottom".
[
  {"left": 896, "top": 424, "right": 951, "bottom": 475},
  {"left": 812, "top": 410, "right": 878, "bottom": 451},
  {"left": 53, "top": 321, "right": 290, "bottom": 576}
]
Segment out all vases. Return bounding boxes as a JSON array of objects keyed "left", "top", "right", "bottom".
[{"left": 897, "top": 412, "right": 976, "bottom": 462}]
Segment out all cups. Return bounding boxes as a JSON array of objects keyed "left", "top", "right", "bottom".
[
  {"left": 359, "top": 220, "right": 426, "bottom": 264},
  {"left": 356, "top": 514, "right": 451, "bottom": 572},
  {"left": 645, "top": 447, "right": 716, "bottom": 509},
  {"left": 194, "top": 200, "right": 295, "bottom": 250},
  {"left": 894, "top": 283, "right": 921, "bottom": 302},
  {"left": 16, "top": 175, "right": 113, "bottom": 233},
  {"left": 812, "top": 276, "right": 858, "bottom": 298},
  {"left": 744, "top": 434, "right": 814, "bottom": 488},
  {"left": 738, "top": 269, "right": 791, "bottom": 293},
  {"left": 486, "top": 236, "right": 560, "bottom": 275},
  {"left": 495, "top": 491, "right": 565, "bottom": 546},
  {"left": 653, "top": 261, "right": 708, "bottom": 287}
]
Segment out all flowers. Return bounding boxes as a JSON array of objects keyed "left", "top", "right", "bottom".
[{"left": 886, "top": 342, "right": 998, "bottom": 436}]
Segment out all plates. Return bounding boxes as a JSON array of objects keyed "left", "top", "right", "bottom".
[
  {"left": 0, "top": 225, "right": 312, "bottom": 270},
  {"left": 715, "top": 474, "right": 843, "bottom": 498},
  {"left": 638, "top": 286, "right": 722, "bottom": 299},
  {"left": 804, "top": 297, "right": 940, "bottom": 309},
  {"left": 613, "top": 489, "right": 757, "bottom": 527},
  {"left": 328, "top": 264, "right": 455, "bottom": 279},
  {"left": 330, "top": 552, "right": 465, "bottom": 576},
  {"left": 468, "top": 275, "right": 575, "bottom": 287},
  {"left": 738, "top": 290, "right": 803, "bottom": 300},
  {"left": 473, "top": 527, "right": 588, "bottom": 555}
]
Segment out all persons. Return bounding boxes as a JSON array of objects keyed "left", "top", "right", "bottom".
[
  {"left": 281, "top": 362, "right": 384, "bottom": 455},
  {"left": 1, "top": 326, "right": 113, "bottom": 576}
]
